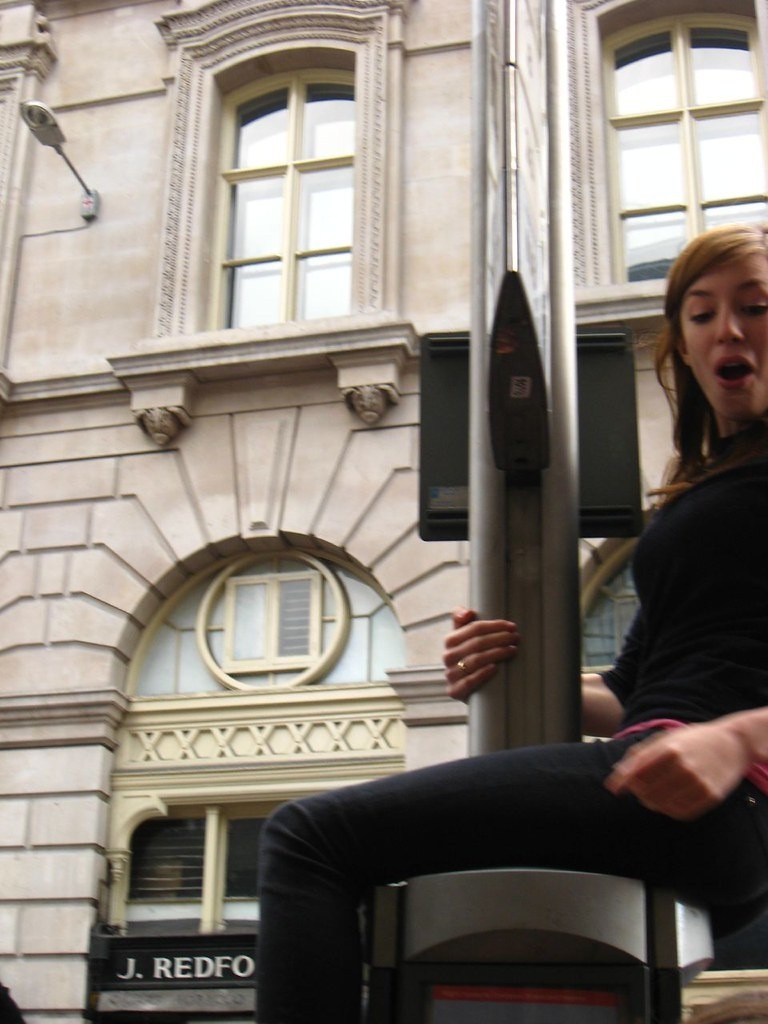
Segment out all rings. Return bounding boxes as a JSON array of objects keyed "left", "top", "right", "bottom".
[{"left": 457, "top": 660, "right": 472, "bottom": 674}]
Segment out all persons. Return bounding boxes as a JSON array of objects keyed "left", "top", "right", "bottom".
[{"left": 251, "top": 219, "right": 768, "bottom": 1024}]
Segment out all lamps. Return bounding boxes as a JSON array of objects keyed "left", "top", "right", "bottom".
[{"left": 20, "top": 99, "right": 103, "bottom": 225}]
